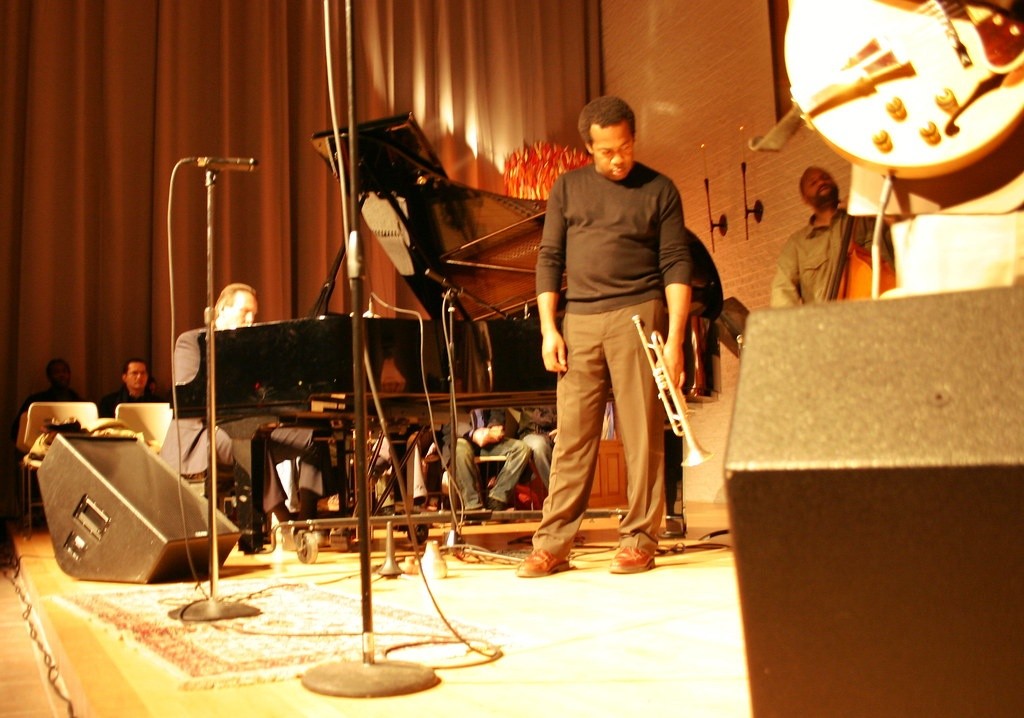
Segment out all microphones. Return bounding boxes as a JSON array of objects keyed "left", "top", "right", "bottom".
[
  {"left": 180, "top": 157, "right": 259, "bottom": 172},
  {"left": 523, "top": 301, "right": 529, "bottom": 322}
]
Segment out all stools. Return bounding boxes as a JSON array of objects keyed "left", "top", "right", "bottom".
[{"left": 184, "top": 464, "right": 246, "bottom": 512}]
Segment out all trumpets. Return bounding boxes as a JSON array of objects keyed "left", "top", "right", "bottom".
[{"left": 632, "top": 313, "right": 714, "bottom": 467}]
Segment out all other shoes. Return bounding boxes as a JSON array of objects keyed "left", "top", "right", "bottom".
[
  {"left": 382, "top": 504, "right": 395, "bottom": 516},
  {"left": 428, "top": 497, "right": 438, "bottom": 512},
  {"left": 488, "top": 496, "right": 506, "bottom": 512}
]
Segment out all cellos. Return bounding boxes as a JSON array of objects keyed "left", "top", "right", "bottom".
[{"left": 817, "top": 214, "right": 895, "bottom": 302}]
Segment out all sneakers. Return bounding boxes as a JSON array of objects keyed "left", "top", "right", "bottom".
[
  {"left": 611, "top": 547, "right": 654, "bottom": 574},
  {"left": 518, "top": 552, "right": 568, "bottom": 579}
]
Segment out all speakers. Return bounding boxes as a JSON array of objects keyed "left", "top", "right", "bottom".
[
  {"left": 724, "top": 283, "right": 1024, "bottom": 718},
  {"left": 36, "top": 433, "right": 242, "bottom": 583}
]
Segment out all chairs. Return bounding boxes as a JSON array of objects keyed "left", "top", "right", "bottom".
[
  {"left": 472, "top": 407, "right": 534, "bottom": 511},
  {"left": 16, "top": 401, "right": 175, "bottom": 539}
]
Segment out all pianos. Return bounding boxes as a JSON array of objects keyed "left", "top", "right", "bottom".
[{"left": 172, "top": 111, "right": 724, "bottom": 555}]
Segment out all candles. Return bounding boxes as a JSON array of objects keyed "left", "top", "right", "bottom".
[
  {"left": 701, "top": 144, "right": 707, "bottom": 179},
  {"left": 740, "top": 126, "right": 744, "bottom": 162}
]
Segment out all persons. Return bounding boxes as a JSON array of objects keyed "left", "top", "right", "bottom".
[
  {"left": 513, "top": 97, "right": 692, "bottom": 577},
  {"left": 664, "top": 227, "right": 724, "bottom": 538},
  {"left": 16, "top": 282, "right": 555, "bottom": 551},
  {"left": 770, "top": 166, "right": 895, "bottom": 309}
]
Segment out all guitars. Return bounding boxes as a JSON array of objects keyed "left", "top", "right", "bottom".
[{"left": 783, "top": 0, "right": 1024, "bottom": 181}]
